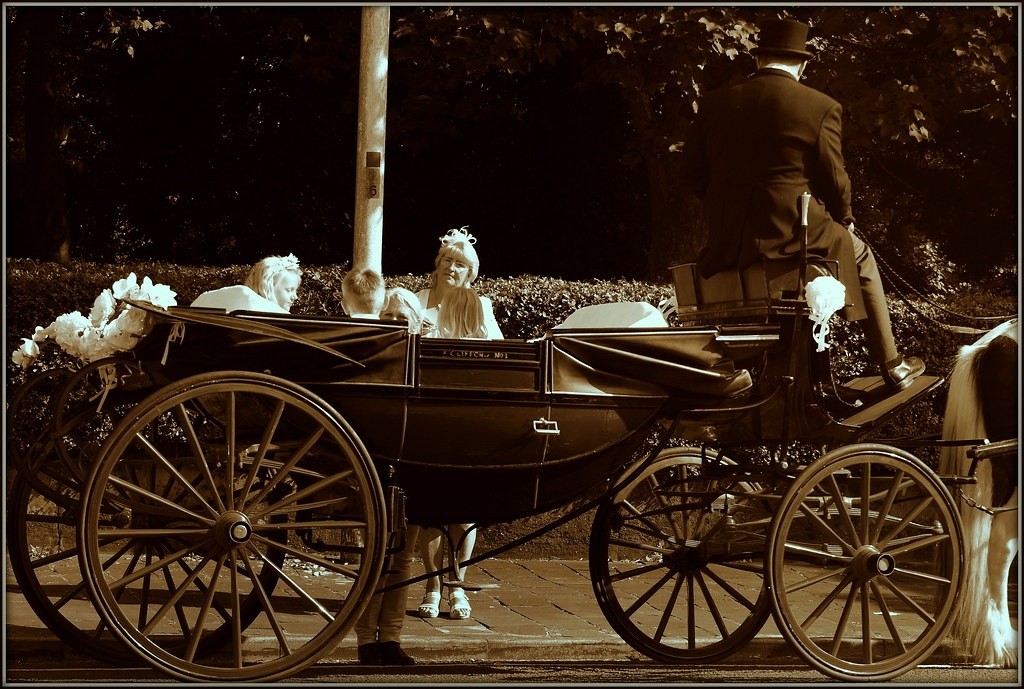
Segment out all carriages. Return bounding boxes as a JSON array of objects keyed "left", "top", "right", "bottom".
[{"left": 5, "top": 251, "right": 1020, "bottom": 688}]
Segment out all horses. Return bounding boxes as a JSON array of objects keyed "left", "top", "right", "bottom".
[{"left": 940, "top": 317, "right": 1021, "bottom": 671}]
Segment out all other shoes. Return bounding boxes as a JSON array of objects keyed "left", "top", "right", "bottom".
[
  {"left": 381, "top": 641, "right": 416, "bottom": 666},
  {"left": 357, "top": 641, "right": 389, "bottom": 668}
]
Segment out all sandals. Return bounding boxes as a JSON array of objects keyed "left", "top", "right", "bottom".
[
  {"left": 445, "top": 588, "right": 474, "bottom": 620},
  {"left": 418, "top": 585, "right": 441, "bottom": 620}
]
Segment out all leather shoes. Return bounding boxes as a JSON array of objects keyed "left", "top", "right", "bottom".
[{"left": 881, "top": 350, "right": 928, "bottom": 393}]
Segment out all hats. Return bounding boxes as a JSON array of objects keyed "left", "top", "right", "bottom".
[{"left": 749, "top": 17, "right": 816, "bottom": 64}]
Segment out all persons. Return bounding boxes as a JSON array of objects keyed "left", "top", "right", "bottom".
[
  {"left": 340, "top": 265, "right": 416, "bottom": 665},
  {"left": 413, "top": 225, "right": 505, "bottom": 621},
  {"left": 378, "top": 286, "right": 425, "bottom": 336},
  {"left": 424, "top": 287, "right": 493, "bottom": 340},
  {"left": 685, "top": 17, "right": 927, "bottom": 394},
  {"left": 243, "top": 251, "right": 304, "bottom": 314}
]
